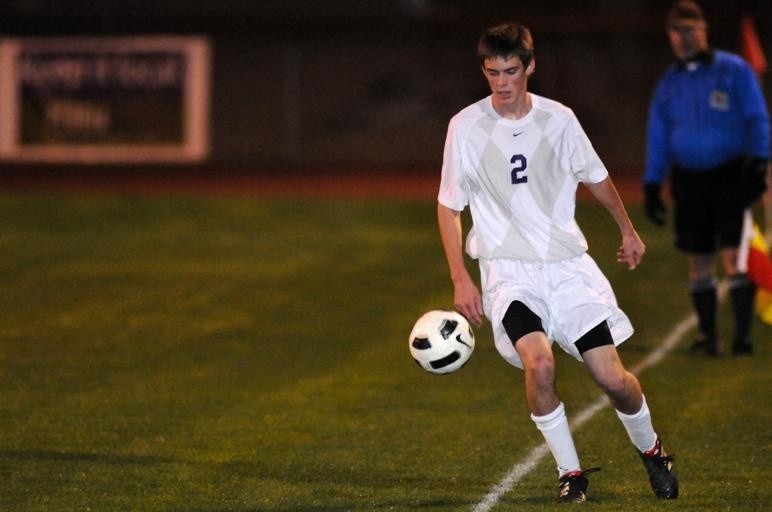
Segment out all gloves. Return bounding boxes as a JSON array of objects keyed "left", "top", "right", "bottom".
[
  {"left": 739, "top": 156, "right": 769, "bottom": 206},
  {"left": 642, "top": 180, "right": 669, "bottom": 227}
]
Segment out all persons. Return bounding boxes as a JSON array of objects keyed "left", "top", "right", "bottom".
[
  {"left": 435, "top": 20, "right": 686, "bottom": 509},
  {"left": 640, "top": 5, "right": 770, "bottom": 364}
]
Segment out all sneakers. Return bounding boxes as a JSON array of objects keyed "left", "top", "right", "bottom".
[
  {"left": 552, "top": 473, "right": 590, "bottom": 504},
  {"left": 689, "top": 337, "right": 720, "bottom": 359},
  {"left": 636, "top": 439, "right": 680, "bottom": 500},
  {"left": 731, "top": 337, "right": 754, "bottom": 358}
]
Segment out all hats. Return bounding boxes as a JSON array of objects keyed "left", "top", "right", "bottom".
[{"left": 665, "top": 1, "right": 703, "bottom": 22}]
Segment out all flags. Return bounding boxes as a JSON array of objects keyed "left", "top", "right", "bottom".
[{"left": 736, "top": 207, "right": 770, "bottom": 328}]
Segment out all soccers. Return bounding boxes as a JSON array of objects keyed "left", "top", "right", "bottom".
[{"left": 409, "top": 309, "right": 476, "bottom": 374}]
